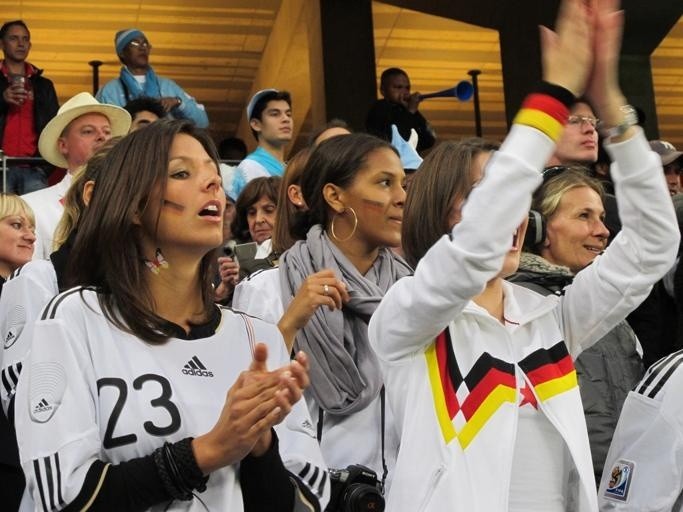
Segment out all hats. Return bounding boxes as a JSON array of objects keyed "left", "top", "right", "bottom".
[
  {"left": 114, "top": 29, "right": 144, "bottom": 53},
  {"left": 648, "top": 139, "right": 682, "bottom": 166},
  {"left": 37, "top": 90, "right": 132, "bottom": 168},
  {"left": 246, "top": 88, "right": 278, "bottom": 122}
]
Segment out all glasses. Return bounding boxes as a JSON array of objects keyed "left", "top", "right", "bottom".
[
  {"left": 130, "top": 42, "right": 152, "bottom": 50},
  {"left": 568, "top": 115, "right": 598, "bottom": 127}
]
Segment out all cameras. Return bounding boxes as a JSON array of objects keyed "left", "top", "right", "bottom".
[
  {"left": 325, "top": 464, "right": 386, "bottom": 512},
  {"left": 222, "top": 237, "right": 260, "bottom": 263}
]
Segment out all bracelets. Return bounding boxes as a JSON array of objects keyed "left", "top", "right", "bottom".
[{"left": 176, "top": 96, "right": 181, "bottom": 106}]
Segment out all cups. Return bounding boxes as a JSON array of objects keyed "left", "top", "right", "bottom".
[{"left": 11, "top": 75, "right": 27, "bottom": 105}]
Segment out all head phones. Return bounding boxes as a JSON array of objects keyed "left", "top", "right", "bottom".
[{"left": 525, "top": 164, "right": 568, "bottom": 247}]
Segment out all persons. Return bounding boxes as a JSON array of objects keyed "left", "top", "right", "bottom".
[
  {"left": 214, "top": 89, "right": 352, "bottom": 306},
  {"left": 0, "top": 136, "right": 122, "bottom": 511},
  {"left": 95, "top": 26, "right": 210, "bottom": 130},
  {"left": 537, "top": 90, "right": 682, "bottom": 365},
  {"left": 0, "top": 20, "right": 59, "bottom": 193},
  {"left": 366, "top": 2, "right": 682, "bottom": 512},
  {"left": 0, "top": 94, "right": 167, "bottom": 295},
  {"left": 12, "top": 118, "right": 330, "bottom": 512},
  {"left": 502, "top": 165, "right": 647, "bottom": 479},
  {"left": 596, "top": 195, "right": 681, "bottom": 512},
  {"left": 235, "top": 135, "right": 414, "bottom": 512},
  {"left": 374, "top": 68, "right": 436, "bottom": 154}
]
